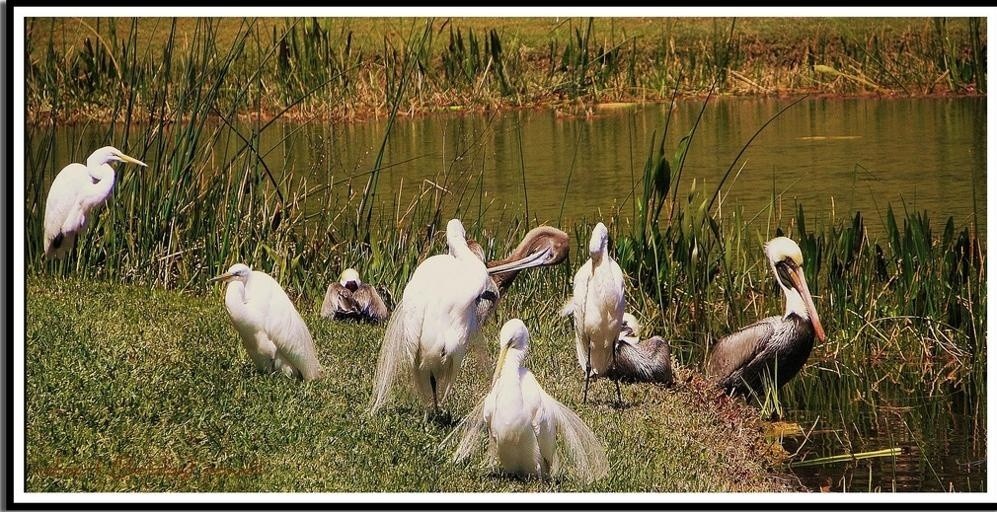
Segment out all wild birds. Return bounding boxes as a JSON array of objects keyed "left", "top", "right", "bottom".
[
  {"left": 206, "top": 262, "right": 325, "bottom": 384},
  {"left": 363, "top": 218, "right": 492, "bottom": 434},
  {"left": 437, "top": 318, "right": 613, "bottom": 487},
  {"left": 467, "top": 223, "right": 570, "bottom": 329},
  {"left": 614, "top": 311, "right": 674, "bottom": 389},
  {"left": 41, "top": 145, "right": 150, "bottom": 282},
  {"left": 705, "top": 234, "right": 825, "bottom": 401},
  {"left": 319, "top": 265, "right": 390, "bottom": 325},
  {"left": 558, "top": 221, "right": 627, "bottom": 409}
]
